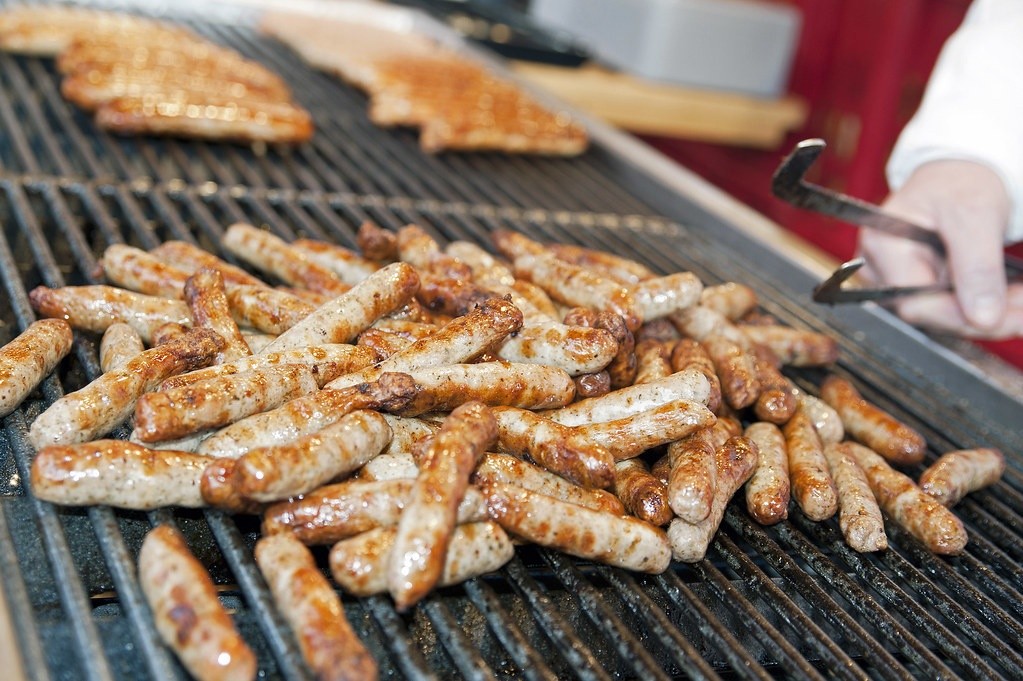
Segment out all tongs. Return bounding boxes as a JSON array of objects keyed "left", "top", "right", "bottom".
[{"left": 772, "top": 137, "right": 1023, "bottom": 304}]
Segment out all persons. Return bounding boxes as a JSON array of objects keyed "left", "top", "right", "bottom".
[{"left": 853, "top": 1, "right": 1023, "bottom": 339}]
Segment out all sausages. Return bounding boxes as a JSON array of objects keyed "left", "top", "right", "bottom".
[{"left": 1, "top": 36, "right": 1007, "bottom": 681}]
